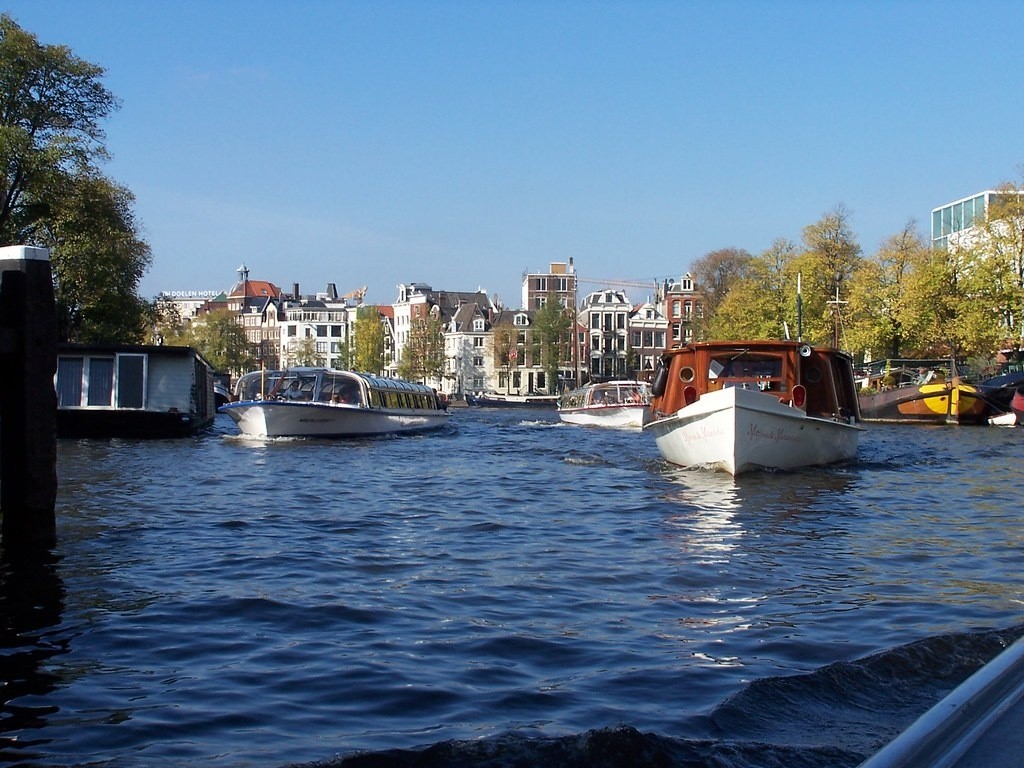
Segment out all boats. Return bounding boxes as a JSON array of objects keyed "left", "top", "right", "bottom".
[
  {"left": 216, "top": 328, "right": 454, "bottom": 437},
  {"left": 557, "top": 269, "right": 1024, "bottom": 474},
  {"left": 464, "top": 390, "right": 559, "bottom": 409},
  {"left": 52, "top": 343, "right": 234, "bottom": 437}
]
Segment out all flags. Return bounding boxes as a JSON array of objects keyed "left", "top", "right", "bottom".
[{"left": 509, "top": 351, "right": 518, "bottom": 360}]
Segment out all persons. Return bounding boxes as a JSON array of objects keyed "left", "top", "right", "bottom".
[
  {"left": 882, "top": 382, "right": 898, "bottom": 394},
  {"left": 916, "top": 367, "right": 937, "bottom": 385},
  {"left": 282, "top": 380, "right": 303, "bottom": 402}
]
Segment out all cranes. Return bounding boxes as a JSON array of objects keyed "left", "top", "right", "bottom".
[{"left": 564, "top": 274, "right": 658, "bottom": 300}]
